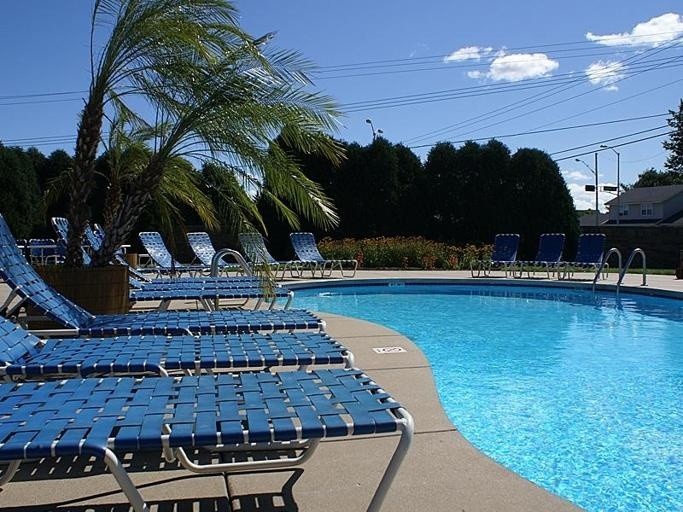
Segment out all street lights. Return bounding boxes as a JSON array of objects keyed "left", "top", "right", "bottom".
[
  {"left": 599, "top": 144, "right": 620, "bottom": 226},
  {"left": 364, "top": 118, "right": 384, "bottom": 142},
  {"left": 574, "top": 152, "right": 599, "bottom": 226}
]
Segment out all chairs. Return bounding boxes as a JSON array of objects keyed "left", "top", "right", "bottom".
[
  {"left": 186, "top": 230, "right": 255, "bottom": 277},
  {"left": 137, "top": 231, "right": 210, "bottom": 279},
  {"left": 23, "top": 236, "right": 133, "bottom": 264},
  {"left": 0, "top": 213, "right": 328, "bottom": 338},
  {"left": 0, "top": 368, "right": 415, "bottom": 511},
  {"left": 115, "top": 253, "right": 266, "bottom": 282},
  {"left": 49, "top": 215, "right": 295, "bottom": 334},
  {"left": 289, "top": 230, "right": 358, "bottom": 278},
  {"left": 0, "top": 315, "right": 355, "bottom": 451},
  {"left": 469, "top": 231, "right": 612, "bottom": 282},
  {"left": 129, "top": 274, "right": 284, "bottom": 292},
  {"left": 239, "top": 232, "right": 304, "bottom": 283}
]
